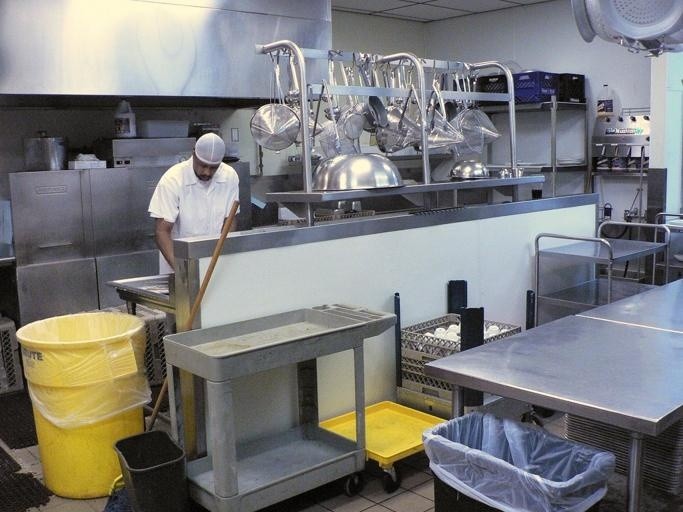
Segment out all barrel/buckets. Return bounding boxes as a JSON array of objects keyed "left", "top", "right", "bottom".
[{"left": 112, "top": 97, "right": 137, "bottom": 139}]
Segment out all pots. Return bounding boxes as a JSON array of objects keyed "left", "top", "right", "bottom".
[
  {"left": 22, "top": 129, "right": 70, "bottom": 172},
  {"left": 437, "top": 72, "right": 479, "bottom": 132},
  {"left": 358, "top": 66, "right": 405, "bottom": 135}
]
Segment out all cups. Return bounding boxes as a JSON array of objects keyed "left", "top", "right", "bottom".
[{"left": 416, "top": 323, "right": 512, "bottom": 354}]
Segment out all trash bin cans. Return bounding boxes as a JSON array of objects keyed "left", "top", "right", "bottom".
[
  {"left": 15, "top": 310, "right": 147, "bottom": 501},
  {"left": 423, "top": 410, "right": 617, "bottom": 512},
  {"left": 115, "top": 429, "right": 187, "bottom": 512}
]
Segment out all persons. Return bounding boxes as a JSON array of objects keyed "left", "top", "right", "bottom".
[{"left": 147, "top": 133, "right": 240, "bottom": 282}]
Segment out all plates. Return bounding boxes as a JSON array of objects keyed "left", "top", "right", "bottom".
[{"left": 516, "top": 158, "right": 585, "bottom": 166}]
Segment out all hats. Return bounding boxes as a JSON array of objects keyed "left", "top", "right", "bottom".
[{"left": 194, "top": 131, "right": 227, "bottom": 165}]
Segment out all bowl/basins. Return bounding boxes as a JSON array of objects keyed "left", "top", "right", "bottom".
[{"left": 496, "top": 168, "right": 524, "bottom": 178}]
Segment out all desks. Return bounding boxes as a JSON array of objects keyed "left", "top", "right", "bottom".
[
  {"left": 574, "top": 276, "right": 683, "bottom": 336},
  {"left": 424, "top": 315, "right": 683, "bottom": 511}
]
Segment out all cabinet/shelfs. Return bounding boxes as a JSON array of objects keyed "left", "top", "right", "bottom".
[
  {"left": 533, "top": 220, "right": 673, "bottom": 327},
  {"left": 469, "top": 100, "right": 593, "bottom": 199},
  {"left": 650, "top": 209, "right": 682, "bottom": 286},
  {"left": 253, "top": 42, "right": 548, "bottom": 228}
]
